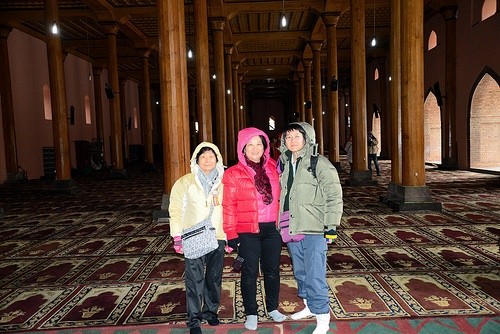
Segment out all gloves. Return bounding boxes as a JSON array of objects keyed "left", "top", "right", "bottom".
[
  {"left": 324, "top": 229, "right": 338, "bottom": 244},
  {"left": 173, "top": 236, "right": 184, "bottom": 254},
  {"left": 228, "top": 238, "right": 241, "bottom": 252},
  {"left": 226, "top": 246, "right": 235, "bottom": 253}
]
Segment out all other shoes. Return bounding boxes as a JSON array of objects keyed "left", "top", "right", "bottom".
[
  {"left": 190, "top": 327, "right": 202, "bottom": 334},
  {"left": 207, "top": 317, "right": 218, "bottom": 326}
]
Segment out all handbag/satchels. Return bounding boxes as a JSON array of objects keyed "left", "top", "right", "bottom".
[
  {"left": 279, "top": 211, "right": 304, "bottom": 243},
  {"left": 181, "top": 218, "right": 219, "bottom": 259},
  {"left": 232, "top": 256, "right": 244, "bottom": 273}
]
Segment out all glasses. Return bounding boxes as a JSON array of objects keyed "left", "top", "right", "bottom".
[{"left": 285, "top": 135, "right": 303, "bottom": 141}]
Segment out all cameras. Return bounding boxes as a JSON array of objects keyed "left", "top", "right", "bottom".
[{"left": 232, "top": 255, "right": 244, "bottom": 270}]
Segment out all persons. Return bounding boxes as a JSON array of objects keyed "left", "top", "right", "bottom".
[
  {"left": 275, "top": 121, "right": 344, "bottom": 334},
  {"left": 367, "top": 130, "right": 382, "bottom": 177},
  {"left": 344, "top": 135, "right": 353, "bottom": 175},
  {"left": 168, "top": 141, "right": 228, "bottom": 334},
  {"left": 222, "top": 127, "right": 288, "bottom": 331}
]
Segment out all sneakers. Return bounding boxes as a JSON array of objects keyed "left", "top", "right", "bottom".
[
  {"left": 268, "top": 310, "right": 286, "bottom": 322},
  {"left": 244, "top": 315, "right": 258, "bottom": 331}
]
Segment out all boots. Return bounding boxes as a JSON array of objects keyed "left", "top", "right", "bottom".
[
  {"left": 312, "top": 311, "right": 330, "bottom": 334},
  {"left": 290, "top": 300, "right": 316, "bottom": 320}
]
